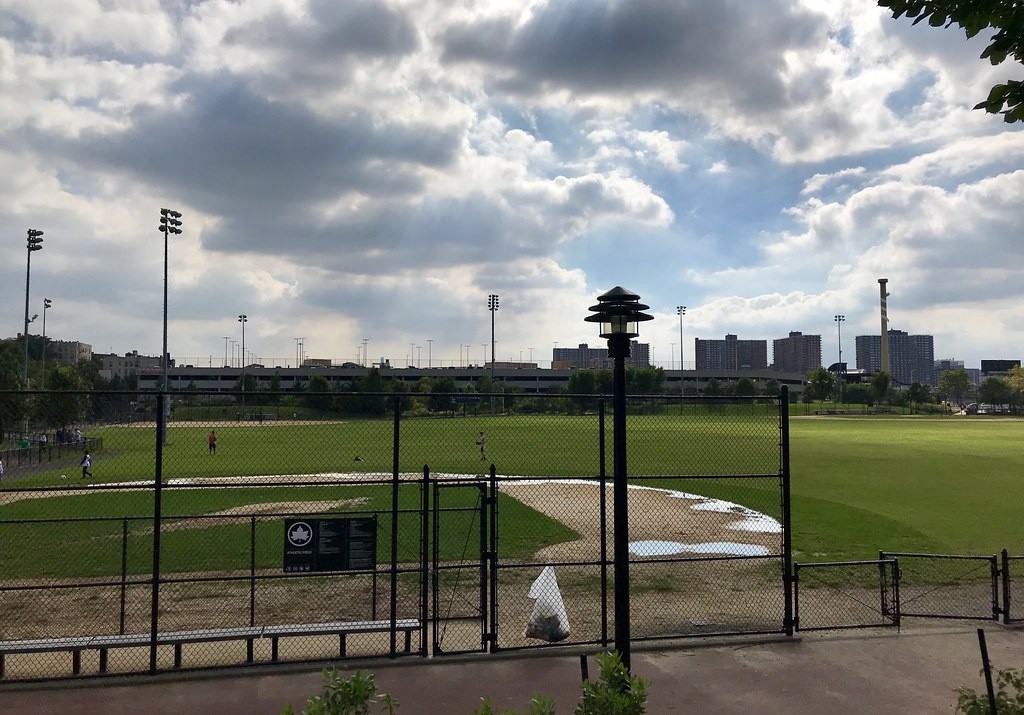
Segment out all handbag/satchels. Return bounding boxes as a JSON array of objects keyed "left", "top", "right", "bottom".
[{"left": 523, "top": 563, "right": 572, "bottom": 642}]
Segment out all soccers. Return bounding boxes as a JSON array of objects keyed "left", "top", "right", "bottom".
[{"left": 60, "top": 475, "right": 67, "bottom": 480}]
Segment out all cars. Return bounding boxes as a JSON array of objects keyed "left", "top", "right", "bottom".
[{"left": 962, "top": 402, "right": 1009, "bottom": 412}]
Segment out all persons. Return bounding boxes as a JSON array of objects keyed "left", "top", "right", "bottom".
[
  {"left": 78, "top": 449, "right": 95, "bottom": 478},
  {"left": 0, "top": 456, "right": 4, "bottom": 479},
  {"left": 55, "top": 426, "right": 83, "bottom": 453},
  {"left": 208, "top": 430, "right": 218, "bottom": 455},
  {"left": 235, "top": 408, "right": 313, "bottom": 432},
  {"left": 39, "top": 432, "right": 48, "bottom": 455},
  {"left": 19, "top": 436, "right": 30, "bottom": 460},
  {"left": 475, "top": 431, "right": 488, "bottom": 461},
  {"left": 355, "top": 455, "right": 365, "bottom": 462},
  {"left": 9, "top": 430, "right": 18, "bottom": 444}
]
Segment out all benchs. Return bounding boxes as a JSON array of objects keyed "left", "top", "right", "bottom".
[
  {"left": 263, "top": 618, "right": 420, "bottom": 663},
  {"left": 88, "top": 626, "right": 264, "bottom": 673},
  {"left": 0, "top": 636, "right": 93, "bottom": 677}
]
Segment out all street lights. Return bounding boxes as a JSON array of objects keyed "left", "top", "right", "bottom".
[
  {"left": 834, "top": 315, "right": 845, "bottom": 379},
  {"left": 159, "top": 207, "right": 182, "bottom": 443},
  {"left": 583, "top": 284, "right": 658, "bottom": 681},
  {"left": 44, "top": 297, "right": 52, "bottom": 352},
  {"left": 24, "top": 229, "right": 43, "bottom": 435},
  {"left": 488, "top": 294, "right": 499, "bottom": 392},
  {"left": 677, "top": 306, "right": 686, "bottom": 394},
  {"left": 238, "top": 314, "right": 247, "bottom": 418},
  {"left": 911, "top": 368, "right": 916, "bottom": 384}
]
